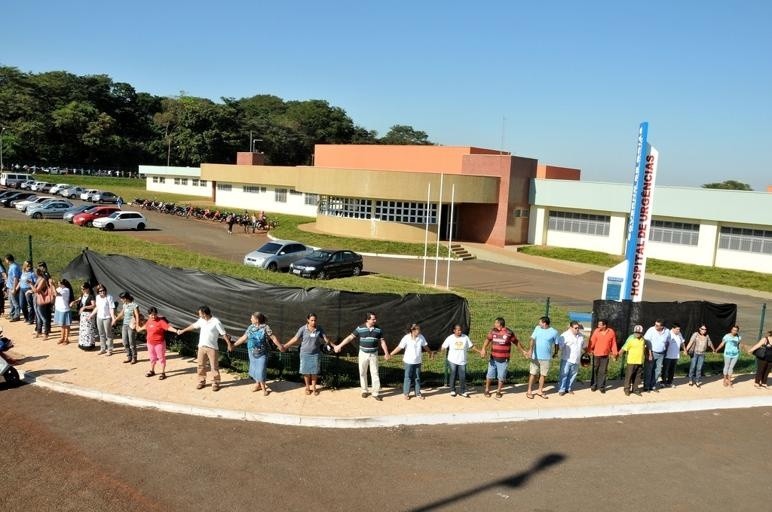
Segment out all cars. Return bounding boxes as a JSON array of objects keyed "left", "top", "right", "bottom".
[
  {"left": 288, "top": 249, "right": 365, "bottom": 280},
  {"left": 0, "top": 168, "right": 147, "bottom": 234},
  {"left": 243, "top": 239, "right": 315, "bottom": 272}
]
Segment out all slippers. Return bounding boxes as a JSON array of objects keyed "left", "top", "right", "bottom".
[{"left": 526, "top": 392, "right": 549, "bottom": 401}]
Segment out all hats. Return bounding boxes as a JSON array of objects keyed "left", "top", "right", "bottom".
[{"left": 633, "top": 325, "right": 645, "bottom": 335}]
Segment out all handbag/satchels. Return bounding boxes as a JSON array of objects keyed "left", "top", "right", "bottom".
[
  {"left": 687, "top": 335, "right": 698, "bottom": 359},
  {"left": 752, "top": 337, "right": 769, "bottom": 359}
]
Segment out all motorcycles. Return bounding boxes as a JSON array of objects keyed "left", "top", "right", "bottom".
[
  {"left": 0, "top": 326, "right": 21, "bottom": 388},
  {"left": 136, "top": 199, "right": 268, "bottom": 231}
]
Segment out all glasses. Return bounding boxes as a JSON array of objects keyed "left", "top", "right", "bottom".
[
  {"left": 574, "top": 326, "right": 580, "bottom": 331},
  {"left": 98, "top": 289, "right": 106, "bottom": 292}
]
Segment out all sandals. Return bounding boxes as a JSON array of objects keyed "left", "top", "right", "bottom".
[
  {"left": 143, "top": 370, "right": 166, "bottom": 380},
  {"left": 688, "top": 379, "right": 703, "bottom": 388}
]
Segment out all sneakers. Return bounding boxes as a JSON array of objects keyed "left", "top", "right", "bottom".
[
  {"left": 196, "top": 380, "right": 221, "bottom": 391},
  {"left": 624, "top": 382, "right": 676, "bottom": 397},
  {"left": 1, "top": 312, "right": 138, "bottom": 365},
  {"left": 590, "top": 384, "right": 606, "bottom": 393},
  {"left": 558, "top": 389, "right": 574, "bottom": 397},
  {"left": 360, "top": 392, "right": 382, "bottom": 401},
  {"left": 484, "top": 390, "right": 502, "bottom": 399},
  {"left": 404, "top": 393, "right": 425, "bottom": 401},
  {"left": 449, "top": 390, "right": 469, "bottom": 398}
]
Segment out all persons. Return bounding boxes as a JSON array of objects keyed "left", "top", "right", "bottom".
[
  {"left": 553, "top": 316, "right": 772, "bottom": 396},
  {"left": 430, "top": 323, "right": 487, "bottom": 397},
  {"left": 524, "top": 316, "right": 559, "bottom": 399},
  {"left": 1, "top": 251, "right": 141, "bottom": 366},
  {"left": 177, "top": 305, "right": 233, "bottom": 391},
  {"left": 384, "top": 324, "right": 434, "bottom": 400},
  {"left": 138, "top": 306, "right": 180, "bottom": 380},
  {"left": 481, "top": 317, "right": 529, "bottom": 398},
  {"left": 334, "top": 311, "right": 389, "bottom": 401},
  {"left": 227, "top": 311, "right": 285, "bottom": 395},
  {"left": 278, "top": 313, "right": 342, "bottom": 395},
  {"left": 226, "top": 211, "right": 267, "bottom": 234}
]
